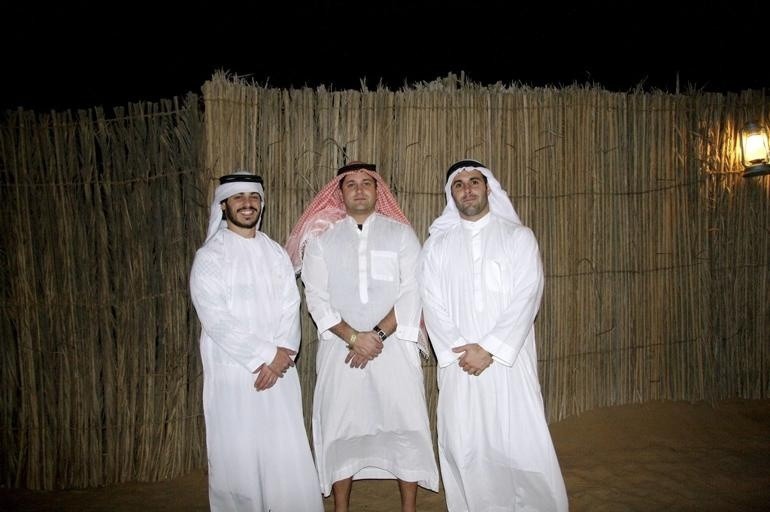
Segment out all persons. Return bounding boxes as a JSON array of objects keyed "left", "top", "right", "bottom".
[
  {"left": 419, "top": 159, "right": 570, "bottom": 512},
  {"left": 284, "top": 160, "right": 441, "bottom": 512},
  {"left": 189, "top": 171, "right": 328, "bottom": 512}
]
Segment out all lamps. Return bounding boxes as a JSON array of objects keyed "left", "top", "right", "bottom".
[{"left": 738, "top": 105, "right": 769, "bottom": 178}]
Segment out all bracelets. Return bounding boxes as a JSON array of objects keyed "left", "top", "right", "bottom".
[
  {"left": 373, "top": 325, "right": 387, "bottom": 341},
  {"left": 349, "top": 330, "right": 358, "bottom": 345}
]
